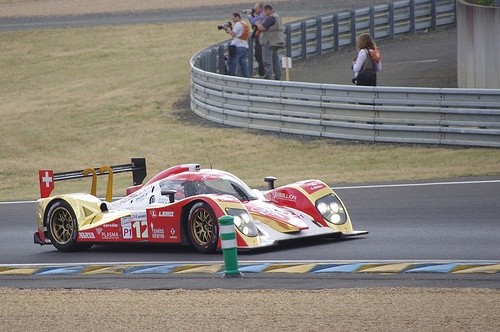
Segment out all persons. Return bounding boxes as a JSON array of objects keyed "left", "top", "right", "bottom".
[
  {"left": 220, "top": 12, "right": 249, "bottom": 79},
  {"left": 255, "top": 4, "right": 285, "bottom": 80},
  {"left": 248, "top": 2, "right": 266, "bottom": 79},
  {"left": 351, "top": 33, "right": 382, "bottom": 87}
]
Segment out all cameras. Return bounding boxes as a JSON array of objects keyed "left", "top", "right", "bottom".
[
  {"left": 217, "top": 22, "right": 232, "bottom": 30},
  {"left": 241, "top": 8, "right": 255, "bottom": 14}
]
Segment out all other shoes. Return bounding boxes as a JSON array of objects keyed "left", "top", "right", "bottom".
[{"left": 253, "top": 74, "right": 262, "bottom": 78}]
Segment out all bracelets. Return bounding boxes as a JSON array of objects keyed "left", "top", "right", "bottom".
[{"left": 247, "top": 15, "right": 251, "bottom": 17}]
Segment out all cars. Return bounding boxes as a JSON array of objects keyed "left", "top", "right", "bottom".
[{"left": 34, "top": 157, "right": 369, "bottom": 257}]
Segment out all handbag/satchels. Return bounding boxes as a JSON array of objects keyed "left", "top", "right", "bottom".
[{"left": 362, "top": 70, "right": 375, "bottom": 85}]
